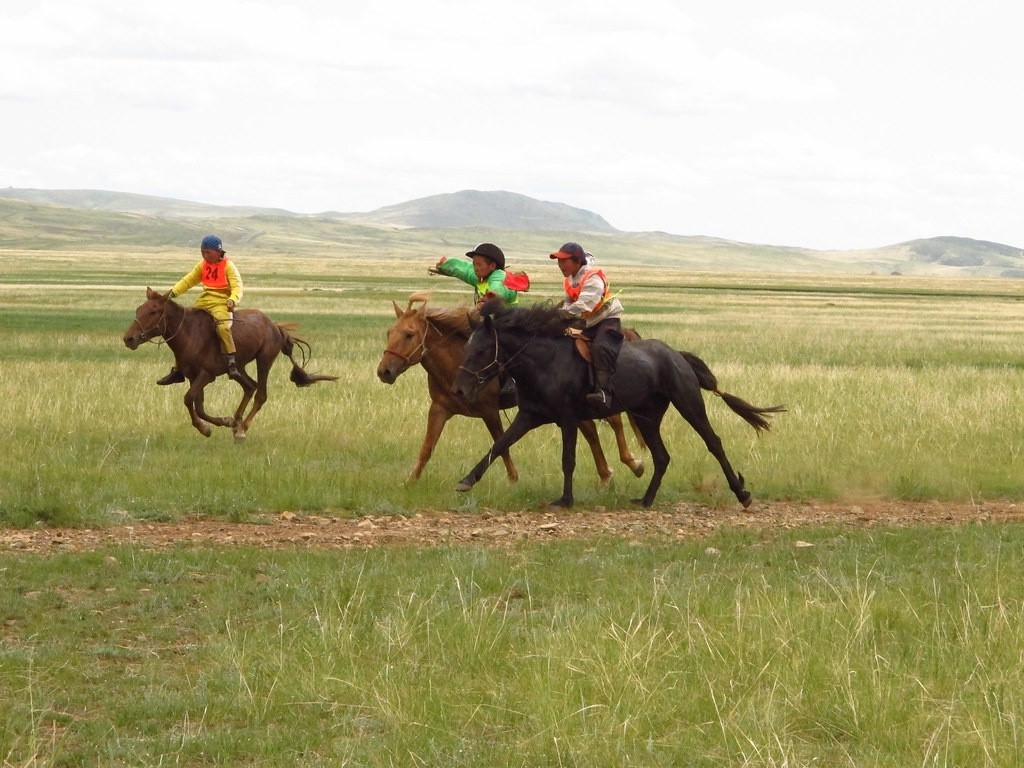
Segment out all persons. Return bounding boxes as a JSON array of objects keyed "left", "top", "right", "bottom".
[
  {"left": 436, "top": 243, "right": 517, "bottom": 322},
  {"left": 550, "top": 242, "right": 624, "bottom": 410},
  {"left": 156, "top": 235, "right": 243, "bottom": 385}
]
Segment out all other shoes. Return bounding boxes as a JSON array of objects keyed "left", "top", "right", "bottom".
[
  {"left": 586, "top": 392, "right": 612, "bottom": 409},
  {"left": 500, "top": 377, "right": 516, "bottom": 394}
]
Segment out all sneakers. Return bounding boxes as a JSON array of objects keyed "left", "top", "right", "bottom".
[
  {"left": 157, "top": 367, "right": 185, "bottom": 385},
  {"left": 228, "top": 363, "right": 242, "bottom": 379}
]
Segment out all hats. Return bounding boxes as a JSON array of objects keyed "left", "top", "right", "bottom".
[
  {"left": 550, "top": 243, "right": 586, "bottom": 258},
  {"left": 466, "top": 243, "right": 505, "bottom": 269},
  {"left": 201, "top": 235, "right": 222, "bottom": 249}
]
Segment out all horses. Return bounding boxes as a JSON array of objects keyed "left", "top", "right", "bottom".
[
  {"left": 123, "top": 287, "right": 341, "bottom": 443},
  {"left": 377, "top": 293, "right": 788, "bottom": 510}
]
